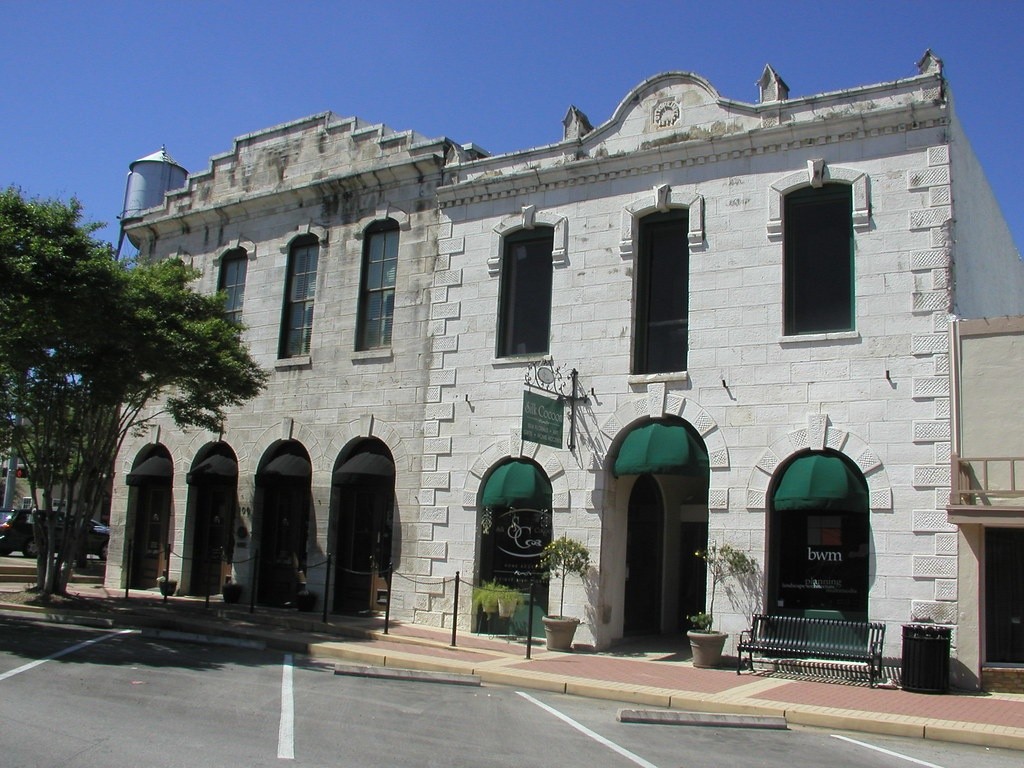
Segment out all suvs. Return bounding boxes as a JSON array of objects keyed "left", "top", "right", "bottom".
[{"left": 0, "top": 507, "right": 69, "bottom": 559}]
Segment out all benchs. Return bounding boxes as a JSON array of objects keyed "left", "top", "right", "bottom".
[{"left": 736, "top": 614, "right": 887, "bottom": 689}]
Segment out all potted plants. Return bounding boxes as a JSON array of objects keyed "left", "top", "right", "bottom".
[
  {"left": 534, "top": 535, "right": 591, "bottom": 652},
  {"left": 299, "top": 590, "right": 319, "bottom": 611},
  {"left": 686, "top": 542, "right": 759, "bottom": 667},
  {"left": 474, "top": 580, "right": 524, "bottom": 618}
]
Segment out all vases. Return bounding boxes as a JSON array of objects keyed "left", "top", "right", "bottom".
[
  {"left": 160, "top": 581, "right": 176, "bottom": 597},
  {"left": 222, "top": 583, "right": 242, "bottom": 604}
]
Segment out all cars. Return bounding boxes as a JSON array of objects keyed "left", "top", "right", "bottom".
[{"left": 67, "top": 515, "right": 109, "bottom": 560}]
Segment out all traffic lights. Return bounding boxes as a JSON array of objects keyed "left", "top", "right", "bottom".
[{"left": 15, "top": 467, "right": 27, "bottom": 478}]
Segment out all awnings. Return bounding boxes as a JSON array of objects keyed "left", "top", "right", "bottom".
[
  {"left": 770, "top": 454, "right": 869, "bottom": 512},
  {"left": 186, "top": 454, "right": 238, "bottom": 482},
  {"left": 126, "top": 456, "right": 172, "bottom": 488},
  {"left": 262, "top": 454, "right": 312, "bottom": 480},
  {"left": 479, "top": 461, "right": 553, "bottom": 512},
  {"left": 335, "top": 451, "right": 394, "bottom": 492},
  {"left": 612, "top": 424, "right": 709, "bottom": 476}
]
